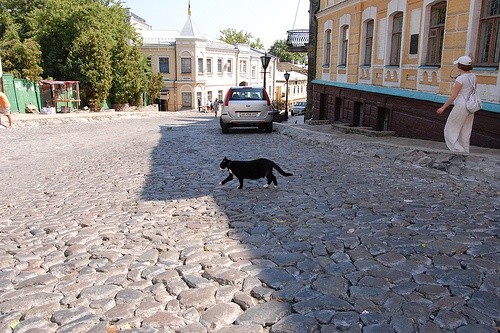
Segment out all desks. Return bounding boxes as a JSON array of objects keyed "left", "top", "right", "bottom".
[{"left": 53, "top": 99, "right": 79, "bottom": 113}]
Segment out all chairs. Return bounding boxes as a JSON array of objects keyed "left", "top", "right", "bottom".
[
  {"left": 252, "top": 94, "right": 258, "bottom": 98},
  {"left": 232, "top": 94, "right": 240, "bottom": 98}
]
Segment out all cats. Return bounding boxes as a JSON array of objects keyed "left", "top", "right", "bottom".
[{"left": 218, "top": 156, "right": 294, "bottom": 189}]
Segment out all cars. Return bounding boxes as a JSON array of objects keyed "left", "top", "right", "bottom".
[
  {"left": 291, "top": 101, "right": 307, "bottom": 116},
  {"left": 220, "top": 86, "right": 274, "bottom": 134}
]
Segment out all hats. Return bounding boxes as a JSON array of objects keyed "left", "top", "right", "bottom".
[{"left": 453, "top": 56, "right": 472, "bottom": 65}]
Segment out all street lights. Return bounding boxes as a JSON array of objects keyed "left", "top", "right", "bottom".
[
  {"left": 260, "top": 52, "right": 271, "bottom": 88},
  {"left": 283, "top": 71, "right": 290, "bottom": 118}
]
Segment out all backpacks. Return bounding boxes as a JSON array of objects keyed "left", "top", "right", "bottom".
[{"left": 460, "top": 75, "right": 482, "bottom": 113}]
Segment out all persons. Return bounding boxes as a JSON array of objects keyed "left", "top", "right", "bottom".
[
  {"left": 198, "top": 98, "right": 223, "bottom": 118},
  {"left": 0, "top": 92, "right": 12, "bottom": 128},
  {"left": 437, "top": 55, "right": 478, "bottom": 156}
]
